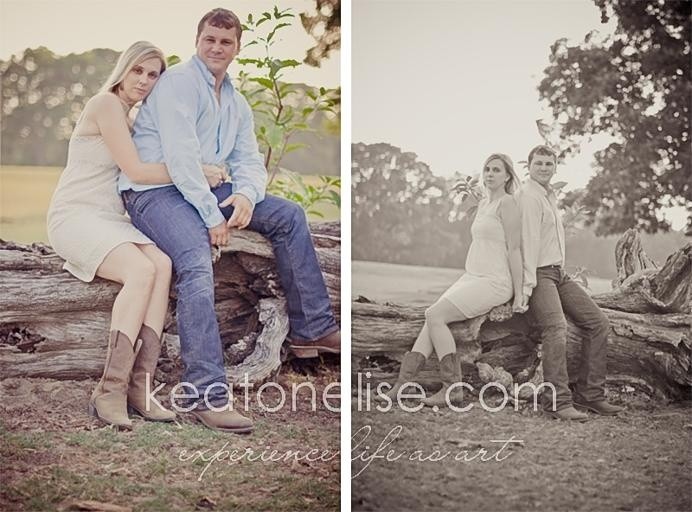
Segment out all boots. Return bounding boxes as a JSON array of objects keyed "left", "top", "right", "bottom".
[
  {"left": 420, "top": 353, "right": 463, "bottom": 408},
  {"left": 127, "top": 323, "right": 176, "bottom": 430},
  {"left": 88, "top": 329, "right": 143, "bottom": 431},
  {"left": 374, "top": 350, "right": 426, "bottom": 402}
]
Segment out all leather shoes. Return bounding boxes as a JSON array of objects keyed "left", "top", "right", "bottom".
[
  {"left": 190, "top": 409, "right": 254, "bottom": 433},
  {"left": 573, "top": 400, "right": 624, "bottom": 416},
  {"left": 547, "top": 406, "right": 589, "bottom": 422},
  {"left": 288, "top": 329, "right": 341, "bottom": 359}
]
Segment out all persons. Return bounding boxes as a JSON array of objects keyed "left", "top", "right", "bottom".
[
  {"left": 46, "top": 6, "right": 340, "bottom": 434},
  {"left": 375, "top": 145, "right": 623, "bottom": 423}
]
